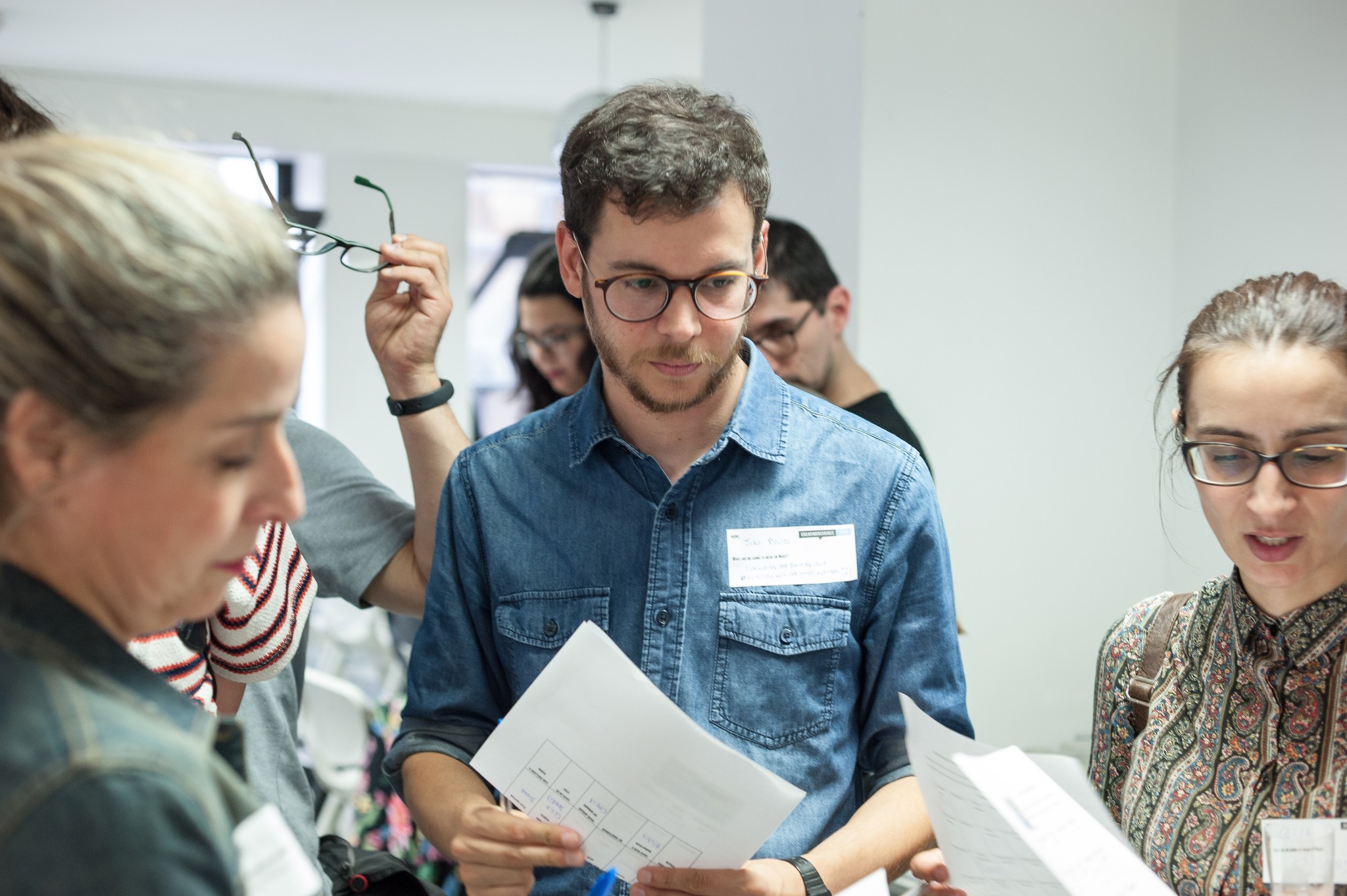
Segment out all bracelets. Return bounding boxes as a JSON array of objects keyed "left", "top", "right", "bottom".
[
  {"left": 387, "top": 377, "right": 454, "bottom": 417},
  {"left": 781, "top": 857, "right": 831, "bottom": 896}
]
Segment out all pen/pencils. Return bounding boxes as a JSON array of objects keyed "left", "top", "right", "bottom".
[
  {"left": 498, "top": 718, "right": 509, "bottom": 810},
  {"left": 592, "top": 866, "right": 619, "bottom": 896}
]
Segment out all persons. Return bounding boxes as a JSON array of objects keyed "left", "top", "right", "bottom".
[
  {"left": 738, "top": 217, "right": 934, "bottom": 484},
  {"left": 236, "top": 233, "right": 473, "bottom": 895},
  {"left": 910, "top": 272, "right": 1347, "bottom": 896},
  {"left": 0, "top": 131, "right": 306, "bottom": 896},
  {"left": 509, "top": 229, "right": 597, "bottom": 411},
  {"left": 0, "top": 80, "right": 319, "bottom": 722},
  {"left": 379, "top": 84, "right": 973, "bottom": 896}
]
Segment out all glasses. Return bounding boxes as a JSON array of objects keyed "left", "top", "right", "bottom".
[
  {"left": 1174, "top": 411, "right": 1347, "bottom": 489},
  {"left": 569, "top": 230, "right": 768, "bottom": 321},
  {"left": 753, "top": 298, "right": 819, "bottom": 359},
  {"left": 513, "top": 319, "right": 586, "bottom": 349},
  {"left": 231, "top": 131, "right": 396, "bottom": 274}
]
